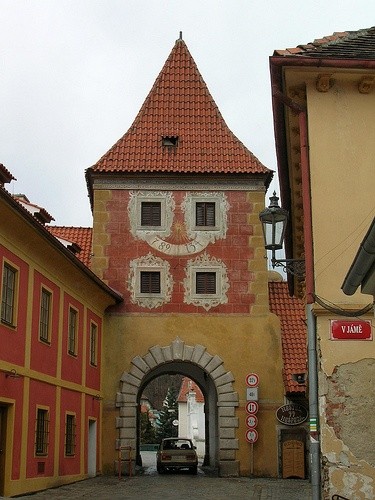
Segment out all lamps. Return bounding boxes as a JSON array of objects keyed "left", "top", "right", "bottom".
[
  {"left": 259, "top": 190, "right": 306, "bottom": 281},
  {"left": 5, "top": 369, "right": 19, "bottom": 378},
  {"left": 92, "top": 393, "right": 103, "bottom": 400}
]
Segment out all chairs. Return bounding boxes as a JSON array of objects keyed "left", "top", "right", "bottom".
[
  {"left": 164, "top": 444, "right": 173, "bottom": 450},
  {"left": 181, "top": 444, "right": 189, "bottom": 449}
]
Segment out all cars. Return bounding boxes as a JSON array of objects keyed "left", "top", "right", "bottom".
[{"left": 154, "top": 437, "right": 199, "bottom": 475}]
